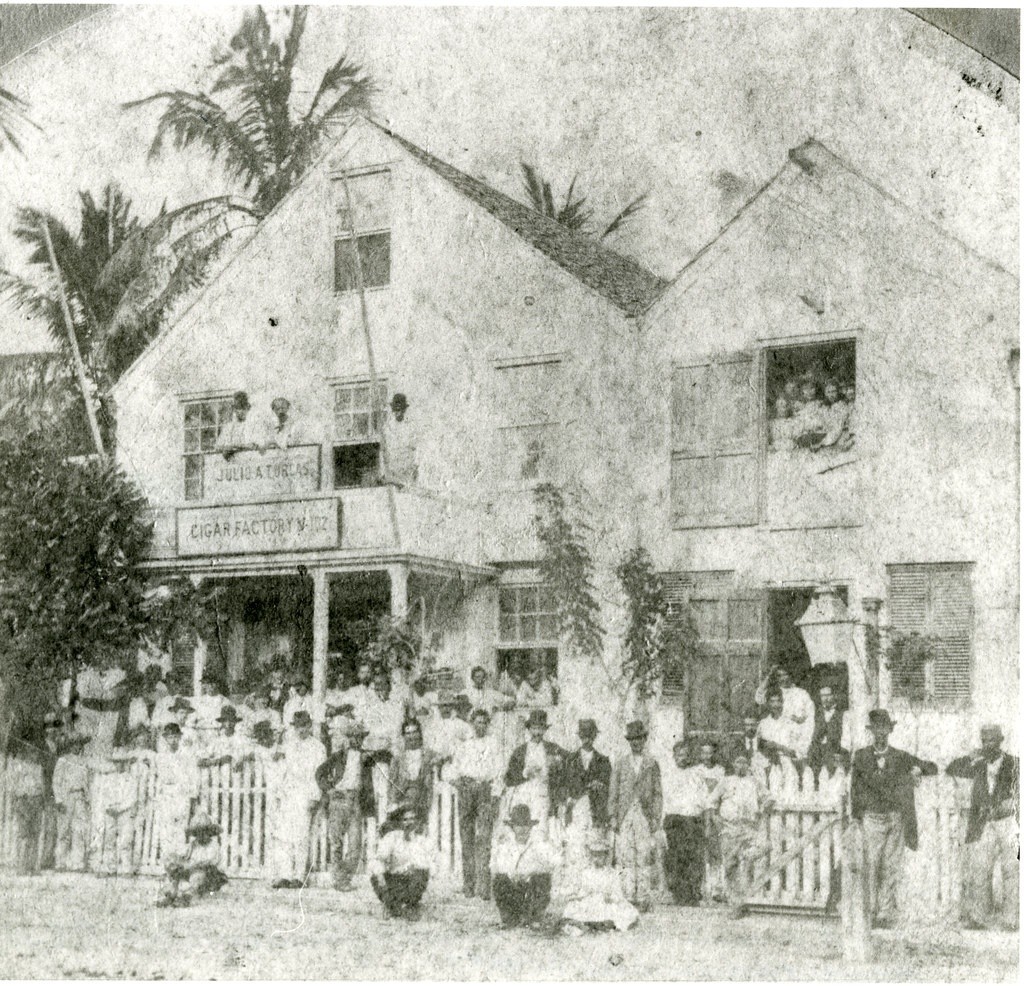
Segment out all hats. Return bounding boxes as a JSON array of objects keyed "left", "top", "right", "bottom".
[
  {"left": 345, "top": 722, "right": 370, "bottom": 738},
  {"left": 523, "top": 710, "right": 551, "bottom": 729},
  {"left": 625, "top": 721, "right": 648, "bottom": 740},
  {"left": 167, "top": 697, "right": 196, "bottom": 713},
  {"left": 289, "top": 711, "right": 314, "bottom": 726},
  {"left": 183, "top": 812, "right": 223, "bottom": 836},
  {"left": 65, "top": 732, "right": 91, "bottom": 747},
  {"left": 864, "top": 709, "right": 897, "bottom": 730},
  {"left": 104, "top": 745, "right": 132, "bottom": 761},
  {"left": 254, "top": 720, "right": 275, "bottom": 738},
  {"left": 503, "top": 805, "right": 540, "bottom": 826},
  {"left": 214, "top": 705, "right": 242, "bottom": 721},
  {"left": 453, "top": 694, "right": 473, "bottom": 711},
  {"left": 574, "top": 719, "right": 601, "bottom": 734},
  {"left": 161, "top": 722, "right": 182, "bottom": 737},
  {"left": 431, "top": 688, "right": 458, "bottom": 706}
]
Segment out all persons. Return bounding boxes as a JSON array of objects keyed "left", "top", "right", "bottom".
[
  {"left": 850, "top": 709, "right": 938, "bottom": 929},
  {"left": 767, "top": 373, "right": 856, "bottom": 451},
  {"left": 378, "top": 394, "right": 418, "bottom": 486},
  {"left": 214, "top": 390, "right": 300, "bottom": 458},
  {"left": 153, "top": 810, "right": 227, "bottom": 907},
  {"left": 946, "top": 725, "right": 1020, "bottom": 932},
  {"left": 370, "top": 808, "right": 439, "bottom": 922},
  {"left": 4, "top": 655, "right": 859, "bottom": 938}
]
